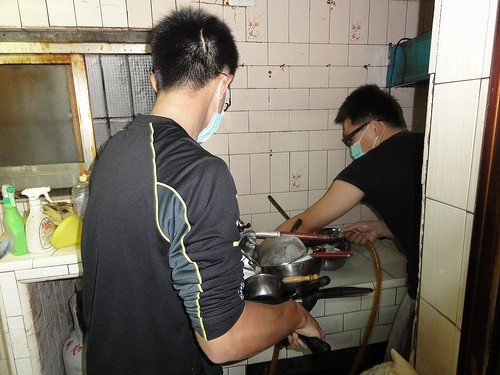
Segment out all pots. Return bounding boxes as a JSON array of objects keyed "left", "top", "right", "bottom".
[{"left": 244, "top": 273, "right": 376, "bottom": 360}]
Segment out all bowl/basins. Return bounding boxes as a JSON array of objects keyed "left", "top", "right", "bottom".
[
  {"left": 301, "top": 238, "right": 352, "bottom": 270},
  {"left": 0, "top": 231, "right": 10, "bottom": 259},
  {"left": 250, "top": 246, "right": 327, "bottom": 279},
  {"left": 315, "top": 227, "right": 344, "bottom": 236}
]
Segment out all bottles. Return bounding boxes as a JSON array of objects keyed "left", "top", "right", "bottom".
[{"left": 71, "top": 173, "right": 89, "bottom": 217}]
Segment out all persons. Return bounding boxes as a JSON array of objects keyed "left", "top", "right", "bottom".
[
  {"left": 81, "top": 10, "right": 326, "bottom": 375},
  {"left": 261, "top": 85, "right": 424, "bottom": 363}
]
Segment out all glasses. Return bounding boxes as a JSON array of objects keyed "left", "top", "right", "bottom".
[
  {"left": 217, "top": 72, "right": 232, "bottom": 111},
  {"left": 342, "top": 118, "right": 382, "bottom": 148}
]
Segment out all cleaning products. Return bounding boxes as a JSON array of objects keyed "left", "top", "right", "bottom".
[
  {"left": 21, "top": 186, "right": 56, "bottom": 254},
  {"left": 1, "top": 184, "right": 28, "bottom": 256}
]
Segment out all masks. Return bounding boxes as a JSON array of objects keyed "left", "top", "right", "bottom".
[
  {"left": 348, "top": 122, "right": 379, "bottom": 160},
  {"left": 196, "top": 100, "right": 223, "bottom": 145}
]
